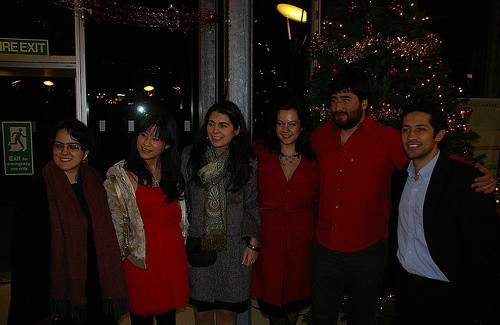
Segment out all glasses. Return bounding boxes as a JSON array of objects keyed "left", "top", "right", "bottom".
[{"left": 51, "top": 143, "right": 82, "bottom": 153}]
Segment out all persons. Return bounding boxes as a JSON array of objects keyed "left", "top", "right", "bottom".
[
  {"left": 102, "top": 107, "right": 191, "bottom": 325},
  {"left": 180, "top": 100, "right": 262, "bottom": 325},
  {"left": 309, "top": 72, "right": 497, "bottom": 325},
  {"left": 6, "top": 116, "right": 130, "bottom": 325},
  {"left": 250, "top": 100, "right": 319, "bottom": 325},
  {"left": 383, "top": 100, "right": 500, "bottom": 325}
]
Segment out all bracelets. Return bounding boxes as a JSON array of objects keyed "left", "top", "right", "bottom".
[{"left": 246, "top": 242, "right": 261, "bottom": 252}]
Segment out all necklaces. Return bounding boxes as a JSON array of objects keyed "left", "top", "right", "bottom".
[{"left": 279, "top": 152, "right": 301, "bottom": 165}]
